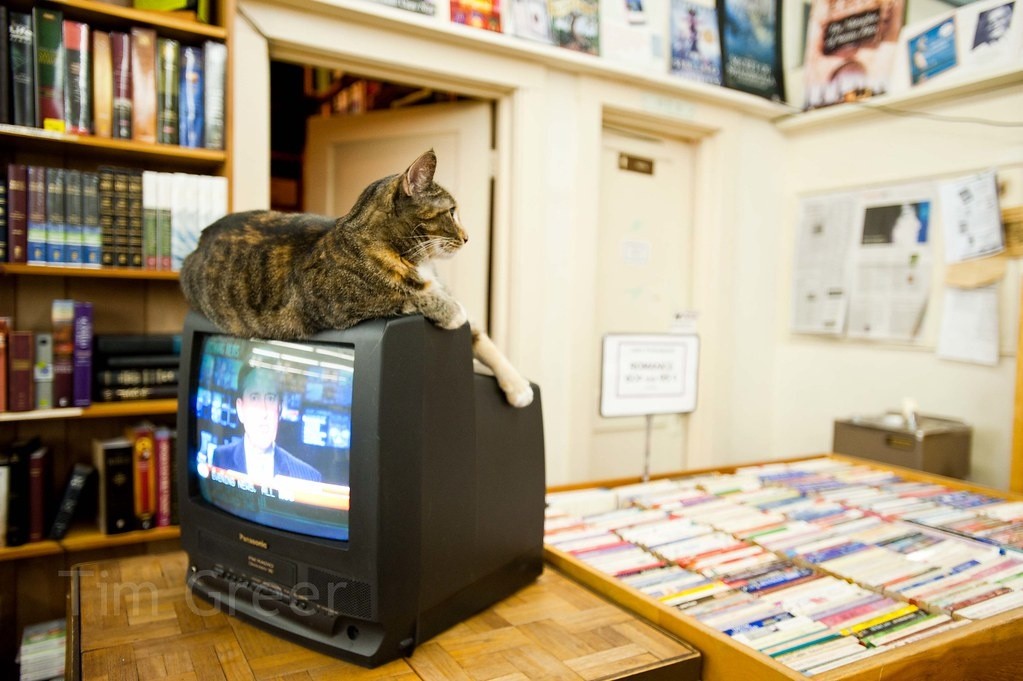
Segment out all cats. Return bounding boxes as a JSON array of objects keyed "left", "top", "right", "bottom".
[{"left": 180, "top": 147, "right": 535, "bottom": 408}]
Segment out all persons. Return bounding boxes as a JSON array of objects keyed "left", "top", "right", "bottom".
[{"left": 208, "top": 360, "right": 323, "bottom": 483}]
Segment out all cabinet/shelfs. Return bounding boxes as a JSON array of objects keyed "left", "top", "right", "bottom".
[{"left": 0, "top": 0, "right": 238, "bottom": 560}]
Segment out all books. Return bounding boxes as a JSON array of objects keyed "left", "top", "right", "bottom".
[
  {"left": 0, "top": 422, "right": 176, "bottom": 545},
  {"left": 386, "top": 0, "right": 1023, "bottom": 111},
  {"left": 16, "top": 616, "right": 66, "bottom": 681},
  {"left": 0, "top": 162, "right": 228, "bottom": 271},
  {"left": 542, "top": 485, "right": 973, "bottom": 675},
  {"left": 0, "top": 0, "right": 225, "bottom": 150},
  {"left": 0, "top": 301, "right": 185, "bottom": 413},
  {"left": 618, "top": 455, "right": 1022, "bottom": 619}
]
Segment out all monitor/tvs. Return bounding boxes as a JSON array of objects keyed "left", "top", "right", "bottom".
[{"left": 177, "top": 307, "right": 546, "bottom": 664}]
